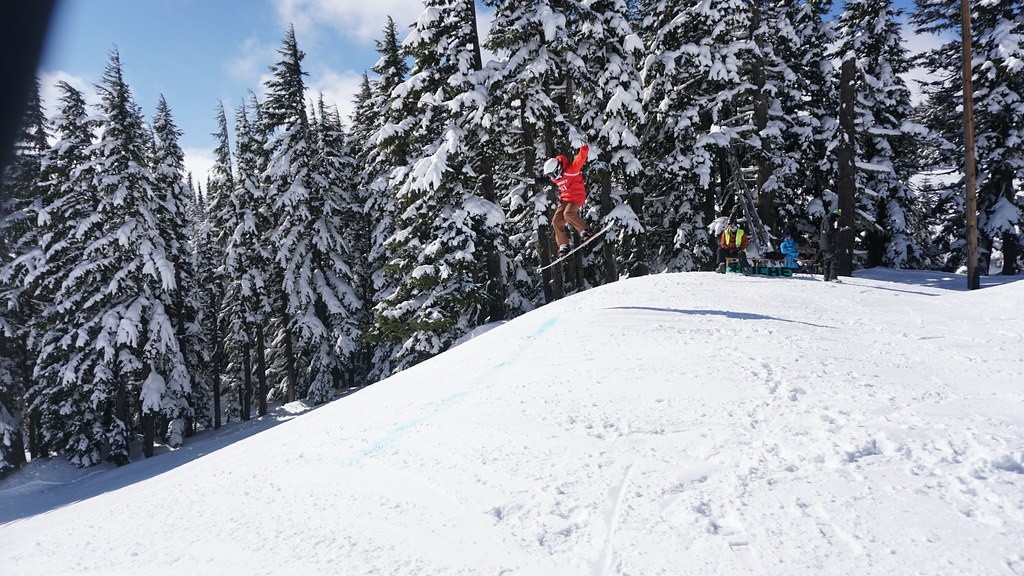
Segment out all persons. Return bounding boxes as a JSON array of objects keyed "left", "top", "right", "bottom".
[
  {"left": 819, "top": 209, "right": 851, "bottom": 284},
  {"left": 542, "top": 134, "right": 592, "bottom": 259},
  {"left": 779, "top": 232, "right": 801, "bottom": 273},
  {"left": 715, "top": 215, "right": 754, "bottom": 276}
]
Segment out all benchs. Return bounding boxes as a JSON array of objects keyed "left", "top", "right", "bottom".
[{"left": 725, "top": 257, "right": 788, "bottom": 273}]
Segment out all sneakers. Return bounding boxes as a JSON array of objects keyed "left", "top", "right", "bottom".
[
  {"left": 557, "top": 245, "right": 570, "bottom": 258},
  {"left": 580, "top": 229, "right": 594, "bottom": 242},
  {"left": 742, "top": 267, "right": 751, "bottom": 276},
  {"left": 716, "top": 264, "right": 725, "bottom": 274}
]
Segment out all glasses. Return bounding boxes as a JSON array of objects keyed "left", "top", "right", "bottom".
[{"left": 728, "top": 222, "right": 734, "bottom": 225}]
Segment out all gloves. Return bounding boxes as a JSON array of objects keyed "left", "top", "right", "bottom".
[{"left": 579, "top": 137, "right": 589, "bottom": 148}]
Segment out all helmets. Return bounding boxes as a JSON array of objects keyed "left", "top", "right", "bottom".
[
  {"left": 544, "top": 158, "right": 565, "bottom": 182},
  {"left": 727, "top": 219, "right": 737, "bottom": 227}
]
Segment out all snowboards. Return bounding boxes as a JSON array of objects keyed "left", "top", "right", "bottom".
[{"left": 532, "top": 220, "right": 615, "bottom": 269}]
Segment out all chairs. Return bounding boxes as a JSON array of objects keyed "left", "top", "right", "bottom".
[{"left": 793, "top": 247, "right": 817, "bottom": 277}]
